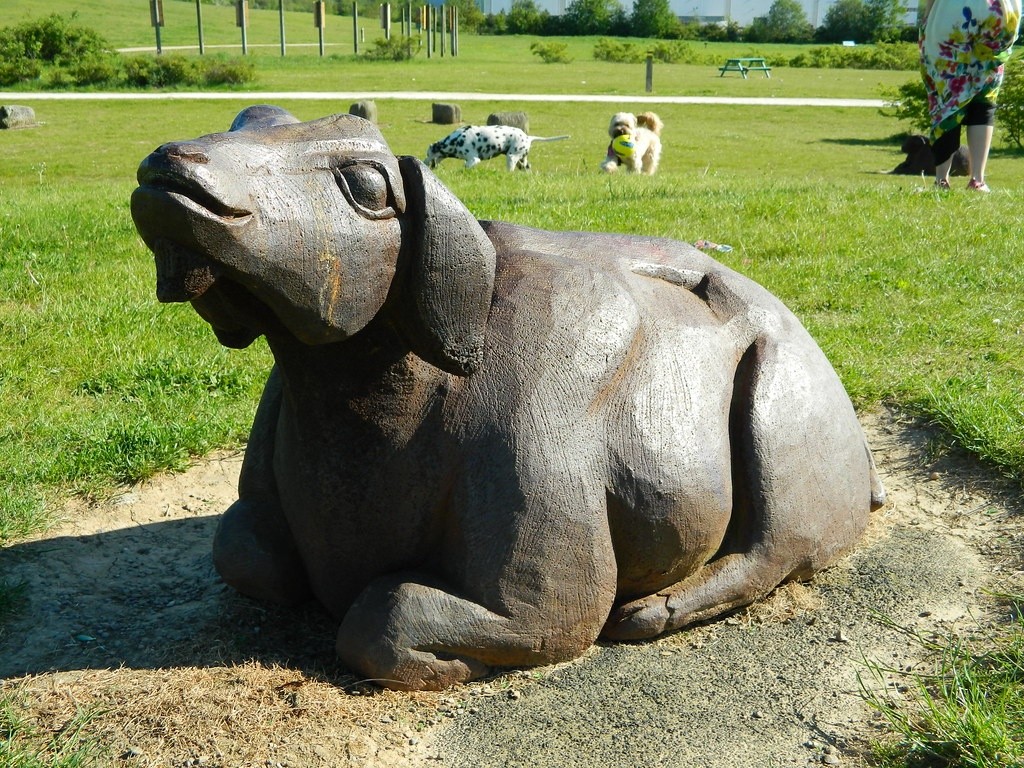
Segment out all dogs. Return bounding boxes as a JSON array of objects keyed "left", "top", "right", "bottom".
[
  {"left": 600, "top": 112, "right": 663, "bottom": 175},
  {"left": 424, "top": 124, "right": 571, "bottom": 173}
]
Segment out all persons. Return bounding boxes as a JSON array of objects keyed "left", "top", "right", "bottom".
[{"left": 915, "top": 0, "right": 1023, "bottom": 192}]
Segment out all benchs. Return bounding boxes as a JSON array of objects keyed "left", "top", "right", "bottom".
[{"left": 718, "top": 66, "right": 772, "bottom": 72}]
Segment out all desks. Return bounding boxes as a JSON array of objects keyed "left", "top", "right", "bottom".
[{"left": 720, "top": 58, "right": 770, "bottom": 79}]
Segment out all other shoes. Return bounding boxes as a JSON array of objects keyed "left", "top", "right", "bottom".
[
  {"left": 966, "top": 178, "right": 991, "bottom": 195},
  {"left": 932, "top": 178, "right": 951, "bottom": 190}
]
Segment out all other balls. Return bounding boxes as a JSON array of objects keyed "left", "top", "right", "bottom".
[{"left": 611, "top": 134, "right": 636, "bottom": 159}]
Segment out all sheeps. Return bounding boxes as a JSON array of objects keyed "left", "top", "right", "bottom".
[{"left": 128, "top": 105, "right": 890, "bottom": 691}]
